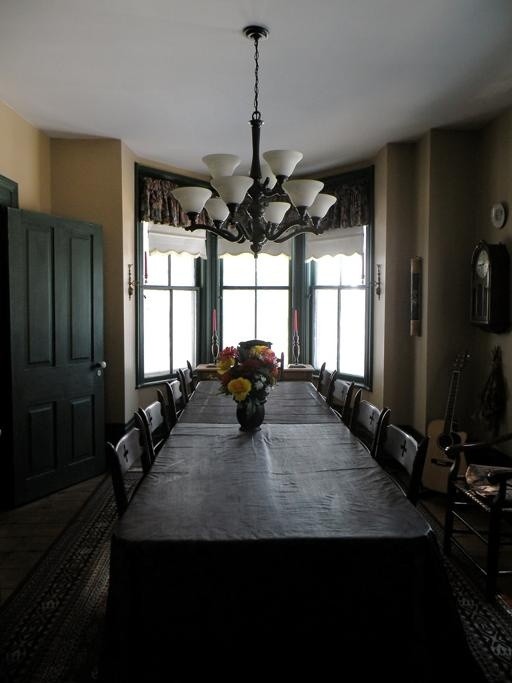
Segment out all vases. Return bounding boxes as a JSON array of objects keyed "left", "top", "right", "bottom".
[
  {"left": 216, "top": 342, "right": 278, "bottom": 399},
  {"left": 236, "top": 398, "right": 265, "bottom": 432}
]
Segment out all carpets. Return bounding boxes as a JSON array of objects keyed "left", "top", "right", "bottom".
[{"left": 0, "top": 468, "right": 512, "bottom": 682}]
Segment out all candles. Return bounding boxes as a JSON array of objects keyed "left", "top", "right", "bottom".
[
  {"left": 292, "top": 308, "right": 298, "bottom": 333},
  {"left": 211, "top": 308, "right": 218, "bottom": 337}
]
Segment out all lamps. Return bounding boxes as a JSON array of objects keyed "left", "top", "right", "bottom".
[{"left": 171, "top": 25, "right": 337, "bottom": 255}]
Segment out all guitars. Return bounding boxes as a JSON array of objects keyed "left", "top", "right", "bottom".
[{"left": 420, "top": 347, "right": 469, "bottom": 494}]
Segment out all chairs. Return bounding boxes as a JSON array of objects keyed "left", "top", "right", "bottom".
[
  {"left": 179, "top": 359, "right": 197, "bottom": 404},
  {"left": 166, "top": 371, "right": 188, "bottom": 423},
  {"left": 374, "top": 408, "right": 428, "bottom": 501},
  {"left": 444, "top": 437, "right": 512, "bottom": 594},
  {"left": 324, "top": 371, "right": 353, "bottom": 421},
  {"left": 316, "top": 362, "right": 337, "bottom": 397},
  {"left": 140, "top": 387, "right": 171, "bottom": 459},
  {"left": 350, "top": 388, "right": 386, "bottom": 455},
  {"left": 276, "top": 352, "right": 285, "bottom": 379},
  {"left": 106, "top": 410, "right": 151, "bottom": 511}
]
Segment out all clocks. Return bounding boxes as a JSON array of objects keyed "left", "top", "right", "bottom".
[{"left": 469, "top": 241, "right": 511, "bottom": 328}]
